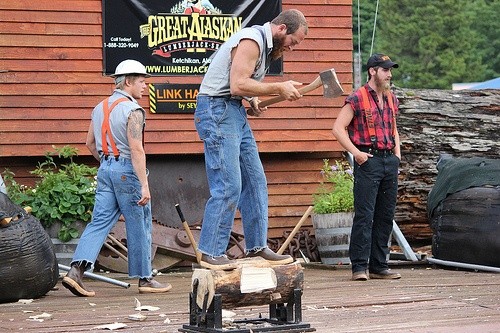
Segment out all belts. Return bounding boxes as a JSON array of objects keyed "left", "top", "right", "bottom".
[{"left": 359, "top": 147, "right": 392, "bottom": 158}]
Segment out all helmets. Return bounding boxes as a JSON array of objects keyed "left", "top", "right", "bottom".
[{"left": 109, "top": 60, "right": 151, "bottom": 78}]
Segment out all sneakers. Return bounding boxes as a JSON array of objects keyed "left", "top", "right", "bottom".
[
  {"left": 200, "top": 251, "right": 237, "bottom": 269},
  {"left": 246, "top": 247, "right": 293, "bottom": 265},
  {"left": 369, "top": 269, "right": 402, "bottom": 279},
  {"left": 352, "top": 271, "right": 367, "bottom": 280}
]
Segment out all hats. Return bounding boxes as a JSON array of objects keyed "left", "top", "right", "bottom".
[{"left": 367, "top": 54, "right": 399, "bottom": 70}]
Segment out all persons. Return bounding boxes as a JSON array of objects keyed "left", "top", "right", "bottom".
[
  {"left": 333, "top": 54, "right": 402, "bottom": 279},
  {"left": 194, "top": 9, "right": 308, "bottom": 269},
  {"left": 62, "top": 60, "right": 172, "bottom": 296}
]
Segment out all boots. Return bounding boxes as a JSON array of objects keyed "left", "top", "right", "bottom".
[
  {"left": 62, "top": 261, "right": 95, "bottom": 297},
  {"left": 138, "top": 278, "right": 171, "bottom": 294}
]
